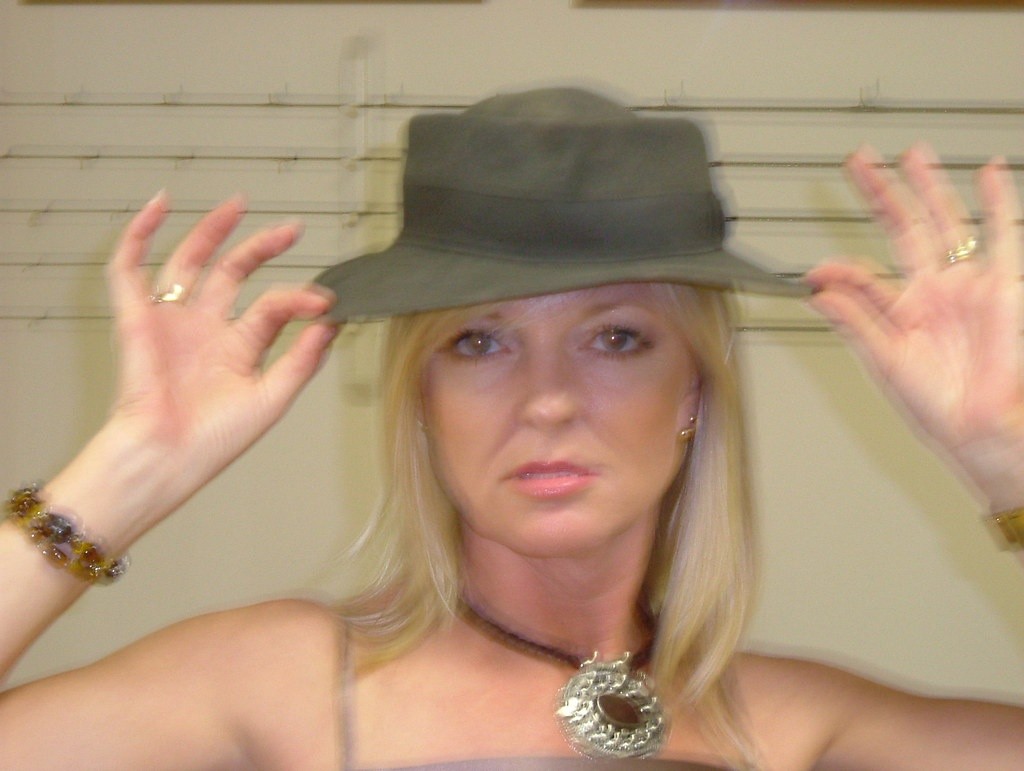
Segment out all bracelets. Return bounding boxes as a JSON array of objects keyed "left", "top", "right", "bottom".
[
  {"left": 10, "top": 488, "right": 128, "bottom": 585},
  {"left": 986, "top": 508, "right": 1024, "bottom": 553}
]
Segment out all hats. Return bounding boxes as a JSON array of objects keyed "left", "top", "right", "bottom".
[{"left": 308, "top": 86, "right": 812, "bottom": 324}]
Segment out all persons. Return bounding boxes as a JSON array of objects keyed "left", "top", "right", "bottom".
[{"left": 0, "top": 88, "right": 1024, "bottom": 771}]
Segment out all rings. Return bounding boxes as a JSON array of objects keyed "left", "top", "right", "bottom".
[
  {"left": 945, "top": 240, "right": 979, "bottom": 265},
  {"left": 147, "top": 293, "right": 181, "bottom": 305}
]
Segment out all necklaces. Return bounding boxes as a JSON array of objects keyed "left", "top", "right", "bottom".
[{"left": 454, "top": 597, "right": 670, "bottom": 760}]
